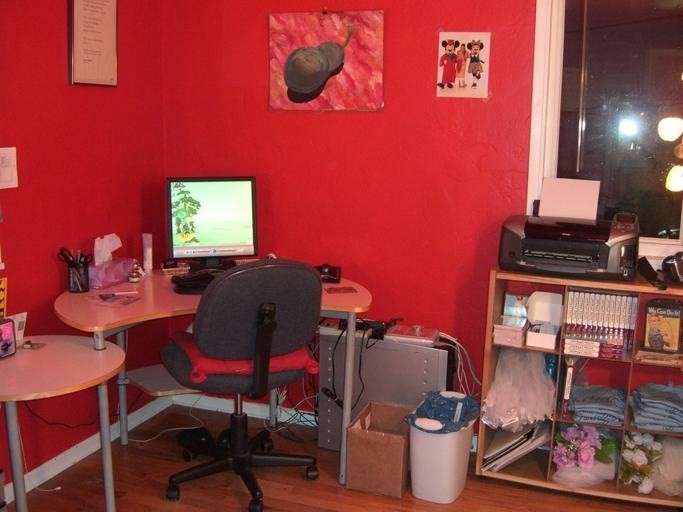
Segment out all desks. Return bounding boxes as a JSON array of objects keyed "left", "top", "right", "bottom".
[
  {"left": 52, "top": 274, "right": 372, "bottom": 488},
  {"left": 0, "top": 333, "right": 124, "bottom": 511}
]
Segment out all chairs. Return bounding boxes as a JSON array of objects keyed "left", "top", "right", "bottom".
[{"left": 163, "top": 256, "right": 322, "bottom": 512}]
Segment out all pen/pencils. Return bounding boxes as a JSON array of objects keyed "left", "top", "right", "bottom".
[{"left": 113, "top": 291, "right": 138, "bottom": 295}]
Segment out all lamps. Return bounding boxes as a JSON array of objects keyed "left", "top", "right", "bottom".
[
  {"left": 656, "top": 114, "right": 683, "bottom": 142},
  {"left": 664, "top": 163, "right": 683, "bottom": 193}
]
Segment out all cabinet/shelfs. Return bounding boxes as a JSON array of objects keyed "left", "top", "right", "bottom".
[{"left": 473, "top": 269, "right": 683, "bottom": 506}]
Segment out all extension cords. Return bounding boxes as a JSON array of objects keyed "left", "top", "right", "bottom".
[{"left": 338, "top": 317, "right": 383, "bottom": 332}]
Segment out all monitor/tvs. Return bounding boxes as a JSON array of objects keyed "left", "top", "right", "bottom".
[{"left": 166, "top": 175, "right": 261, "bottom": 288}]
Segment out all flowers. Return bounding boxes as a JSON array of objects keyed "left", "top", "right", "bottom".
[
  {"left": 552, "top": 422, "right": 617, "bottom": 467},
  {"left": 617, "top": 430, "right": 663, "bottom": 494}
]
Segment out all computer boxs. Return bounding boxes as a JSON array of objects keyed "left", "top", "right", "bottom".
[{"left": 317, "top": 326, "right": 457, "bottom": 453}]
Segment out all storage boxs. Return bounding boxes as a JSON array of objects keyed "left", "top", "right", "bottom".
[{"left": 344, "top": 401, "right": 415, "bottom": 498}]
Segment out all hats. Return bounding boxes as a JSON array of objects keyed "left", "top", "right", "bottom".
[{"left": 283, "top": 40, "right": 344, "bottom": 94}]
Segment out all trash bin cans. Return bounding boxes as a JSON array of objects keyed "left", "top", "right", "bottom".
[{"left": 403, "top": 391, "right": 480, "bottom": 504}]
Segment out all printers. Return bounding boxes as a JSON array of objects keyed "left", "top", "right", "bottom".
[{"left": 498, "top": 199, "right": 639, "bottom": 282}]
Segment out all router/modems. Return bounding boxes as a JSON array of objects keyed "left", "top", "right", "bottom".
[{"left": 384, "top": 323, "right": 440, "bottom": 347}]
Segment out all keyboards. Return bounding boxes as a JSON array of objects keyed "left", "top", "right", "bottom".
[{"left": 172, "top": 283, "right": 210, "bottom": 295}]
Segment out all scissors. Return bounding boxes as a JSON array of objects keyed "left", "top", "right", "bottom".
[
  {"left": 77, "top": 253, "right": 93, "bottom": 267},
  {"left": 57, "top": 248, "right": 80, "bottom": 271}
]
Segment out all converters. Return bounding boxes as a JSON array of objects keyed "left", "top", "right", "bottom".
[{"left": 370, "top": 327, "right": 384, "bottom": 340}]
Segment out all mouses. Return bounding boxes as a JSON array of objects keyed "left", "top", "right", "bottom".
[{"left": 320, "top": 263, "right": 332, "bottom": 275}]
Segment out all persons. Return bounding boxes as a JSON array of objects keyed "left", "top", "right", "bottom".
[{"left": 644, "top": 315, "right": 676, "bottom": 351}]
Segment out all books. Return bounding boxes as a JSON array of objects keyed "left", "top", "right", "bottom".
[
  {"left": 480, "top": 421, "right": 552, "bottom": 473},
  {"left": 561, "top": 290, "right": 636, "bottom": 362},
  {"left": 159, "top": 262, "right": 191, "bottom": 272}
]
